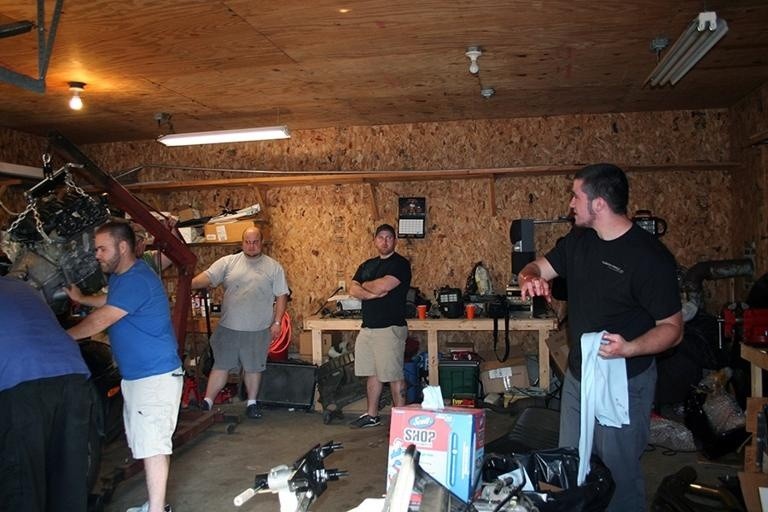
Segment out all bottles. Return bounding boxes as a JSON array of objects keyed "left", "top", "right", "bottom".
[
  {"left": 170, "top": 292, "right": 176, "bottom": 302},
  {"left": 191, "top": 293, "right": 211, "bottom": 318}
]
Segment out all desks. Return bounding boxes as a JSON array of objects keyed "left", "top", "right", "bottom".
[{"left": 303, "top": 311, "right": 559, "bottom": 416}]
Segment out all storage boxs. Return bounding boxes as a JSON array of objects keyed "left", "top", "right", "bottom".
[
  {"left": 203, "top": 217, "right": 269, "bottom": 243},
  {"left": 385, "top": 403, "right": 486, "bottom": 511},
  {"left": 298, "top": 331, "right": 337, "bottom": 355},
  {"left": 438, "top": 346, "right": 530, "bottom": 399}
]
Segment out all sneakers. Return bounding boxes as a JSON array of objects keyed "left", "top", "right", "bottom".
[
  {"left": 349, "top": 413, "right": 381, "bottom": 428},
  {"left": 190, "top": 399, "right": 210, "bottom": 411},
  {"left": 126, "top": 502, "right": 171, "bottom": 512},
  {"left": 246, "top": 403, "right": 262, "bottom": 418}
]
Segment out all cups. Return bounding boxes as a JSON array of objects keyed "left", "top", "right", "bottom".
[
  {"left": 416, "top": 304, "right": 426, "bottom": 319},
  {"left": 465, "top": 304, "right": 477, "bottom": 320}
]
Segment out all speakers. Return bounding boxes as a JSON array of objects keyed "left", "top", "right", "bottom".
[
  {"left": 510, "top": 219, "right": 534, "bottom": 253},
  {"left": 511, "top": 252, "right": 536, "bottom": 285}
]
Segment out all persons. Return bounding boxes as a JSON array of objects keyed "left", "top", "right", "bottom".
[
  {"left": 1, "top": 274, "right": 94, "bottom": 511},
  {"left": 517, "top": 162, "right": 686, "bottom": 512},
  {"left": 127, "top": 216, "right": 187, "bottom": 275},
  {"left": 346, "top": 222, "right": 414, "bottom": 427},
  {"left": 63, "top": 219, "right": 185, "bottom": 512},
  {"left": 191, "top": 226, "right": 292, "bottom": 418}
]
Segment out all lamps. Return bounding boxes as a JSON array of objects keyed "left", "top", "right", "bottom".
[
  {"left": 155, "top": 105, "right": 291, "bottom": 147},
  {"left": 640, "top": 1, "right": 729, "bottom": 91},
  {"left": 464, "top": 48, "right": 483, "bottom": 74},
  {"left": 67, "top": 81, "right": 87, "bottom": 110}
]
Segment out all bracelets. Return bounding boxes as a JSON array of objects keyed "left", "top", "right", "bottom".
[{"left": 273, "top": 321, "right": 283, "bottom": 325}]
